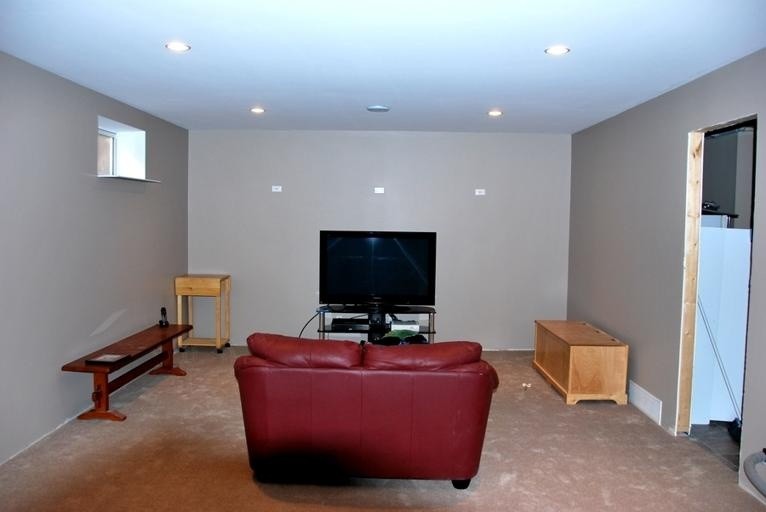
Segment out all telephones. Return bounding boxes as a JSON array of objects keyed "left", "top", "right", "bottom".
[{"left": 159, "top": 307, "right": 169, "bottom": 328}]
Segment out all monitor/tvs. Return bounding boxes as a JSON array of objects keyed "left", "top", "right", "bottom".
[{"left": 320, "top": 230, "right": 436, "bottom": 311}]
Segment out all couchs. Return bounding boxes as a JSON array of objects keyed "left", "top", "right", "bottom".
[{"left": 232, "top": 331, "right": 499, "bottom": 490}]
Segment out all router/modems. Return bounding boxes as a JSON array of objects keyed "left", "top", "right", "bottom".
[{"left": 391, "top": 321, "right": 419, "bottom": 333}]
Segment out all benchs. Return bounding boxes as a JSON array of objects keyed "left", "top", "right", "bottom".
[{"left": 62, "top": 323, "right": 193, "bottom": 421}]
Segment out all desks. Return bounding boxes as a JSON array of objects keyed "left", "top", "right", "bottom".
[
  {"left": 175, "top": 274, "right": 231, "bottom": 353},
  {"left": 317, "top": 305, "right": 437, "bottom": 345}
]
